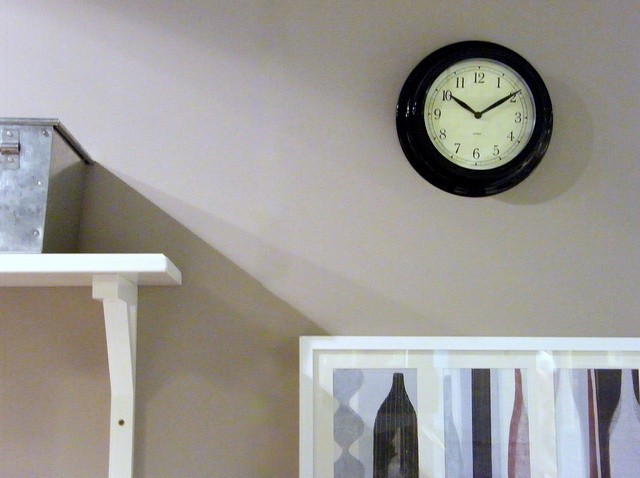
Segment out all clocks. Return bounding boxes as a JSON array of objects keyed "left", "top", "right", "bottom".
[{"left": 396, "top": 40, "right": 553, "bottom": 197}]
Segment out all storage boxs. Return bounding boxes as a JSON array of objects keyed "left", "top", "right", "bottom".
[{"left": 0, "top": 119, "right": 94, "bottom": 252}]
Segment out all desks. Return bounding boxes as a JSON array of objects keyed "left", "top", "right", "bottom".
[{"left": 0, "top": 253, "right": 181, "bottom": 477}]
